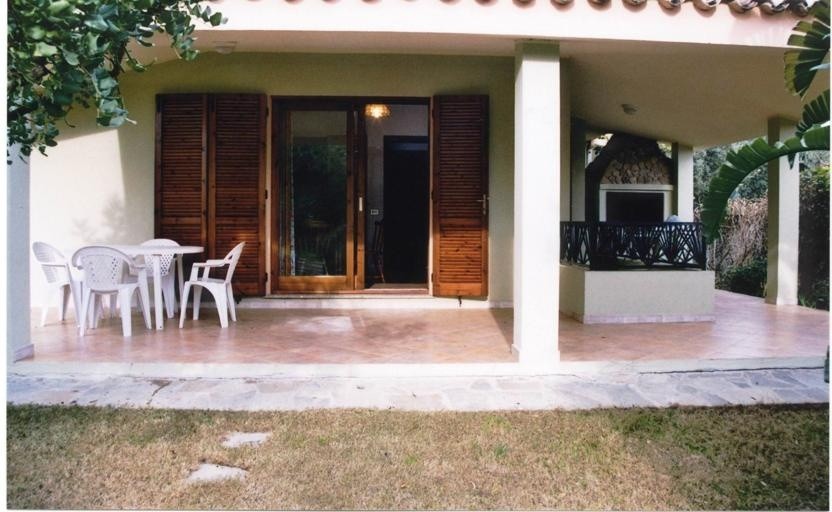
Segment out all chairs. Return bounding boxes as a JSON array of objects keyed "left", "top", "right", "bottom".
[
  {"left": 70, "top": 245, "right": 154, "bottom": 339},
  {"left": 176, "top": 240, "right": 248, "bottom": 331},
  {"left": 126, "top": 237, "right": 186, "bottom": 322},
  {"left": 296, "top": 210, "right": 392, "bottom": 286},
  {"left": 31, "top": 238, "right": 96, "bottom": 325}
]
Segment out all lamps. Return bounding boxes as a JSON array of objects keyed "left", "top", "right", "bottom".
[{"left": 365, "top": 103, "right": 390, "bottom": 119}]
